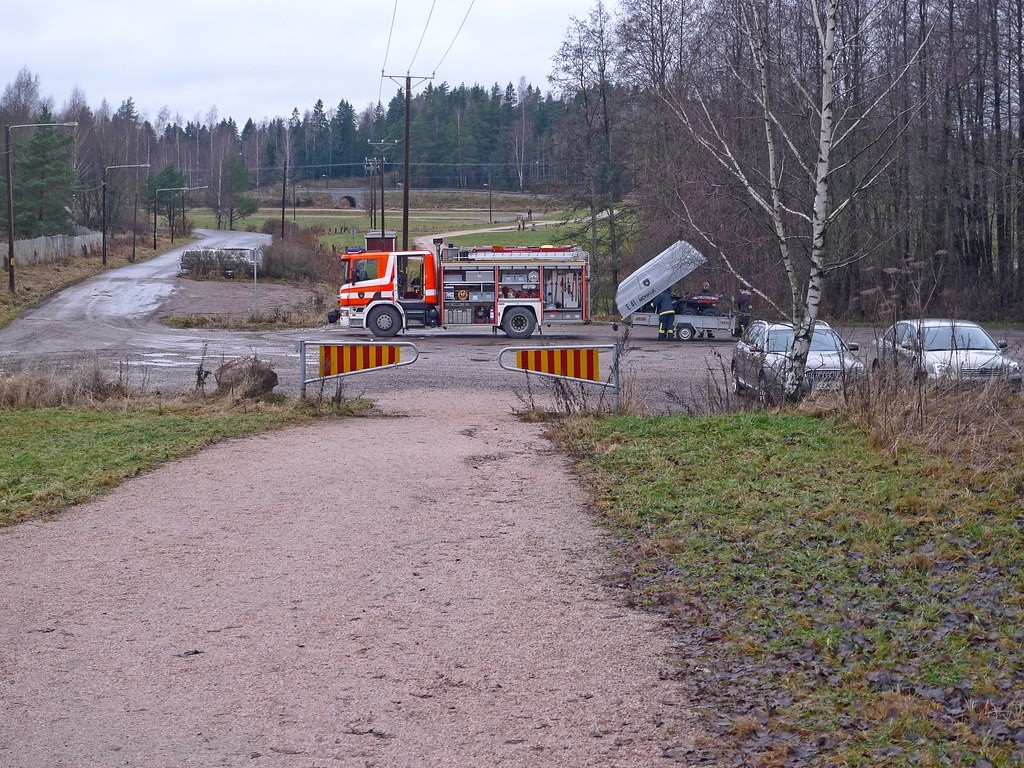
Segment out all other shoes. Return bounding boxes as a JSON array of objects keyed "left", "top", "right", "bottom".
[
  {"left": 698, "top": 333, "right": 703, "bottom": 338},
  {"left": 668, "top": 338, "right": 673, "bottom": 341},
  {"left": 708, "top": 333, "right": 715, "bottom": 338},
  {"left": 657, "top": 338, "right": 664, "bottom": 341}
]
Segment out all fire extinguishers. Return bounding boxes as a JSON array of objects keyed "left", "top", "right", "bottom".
[{"left": 488, "top": 305, "right": 495, "bottom": 324}]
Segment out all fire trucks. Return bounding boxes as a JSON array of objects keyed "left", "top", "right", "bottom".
[{"left": 328, "top": 238, "right": 591, "bottom": 339}]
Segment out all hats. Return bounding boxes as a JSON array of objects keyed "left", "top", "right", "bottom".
[{"left": 740, "top": 285, "right": 748, "bottom": 290}]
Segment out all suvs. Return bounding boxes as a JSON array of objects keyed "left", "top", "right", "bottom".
[{"left": 730, "top": 320, "right": 871, "bottom": 405}]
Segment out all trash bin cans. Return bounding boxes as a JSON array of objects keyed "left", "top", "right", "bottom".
[{"left": 531, "top": 224, "right": 536, "bottom": 231}]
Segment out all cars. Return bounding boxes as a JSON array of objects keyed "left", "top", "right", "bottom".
[{"left": 874, "top": 318, "right": 1023, "bottom": 394}]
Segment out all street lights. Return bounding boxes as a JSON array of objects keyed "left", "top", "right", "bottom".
[
  {"left": 484, "top": 184, "right": 491, "bottom": 217},
  {"left": 182, "top": 185, "right": 209, "bottom": 236},
  {"left": 102, "top": 164, "right": 150, "bottom": 266},
  {"left": 154, "top": 188, "right": 189, "bottom": 251},
  {"left": 323, "top": 175, "right": 328, "bottom": 190},
  {"left": 4, "top": 122, "right": 79, "bottom": 294}
]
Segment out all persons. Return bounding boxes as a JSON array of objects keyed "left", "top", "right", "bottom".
[
  {"left": 518, "top": 220, "right": 521, "bottom": 231},
  {"left": 651, "top": 292, "right": 676, "bottom": 341},
  {"left": 733, "top": 286, "right": 752, "bottom": 336},
  {"left": 698, "top": 282, "right": 716, "bottom": 338}
]
